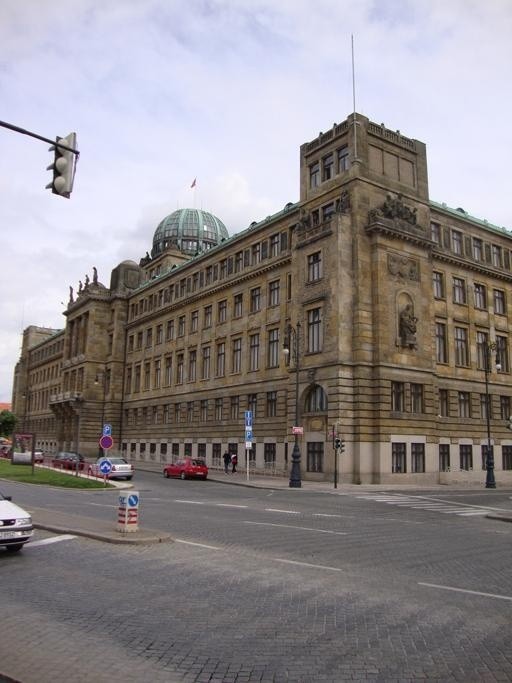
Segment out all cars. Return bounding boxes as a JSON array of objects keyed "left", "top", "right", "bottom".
[
  {"left": 0, "top": 436, "right": 85, "bottom": 470},
  {"left": 0, "top": 486, "right": 35, "bottom": 554},
  {"left": 86, "top": 456, "right": 136, "bottom": 481},
  {"left": 161, "top": 457, "right": 210, "bottom": 481}
]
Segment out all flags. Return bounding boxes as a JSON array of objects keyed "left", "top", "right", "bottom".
[{"left": 190, "top": 177, "right": 196, "bottom": 187}]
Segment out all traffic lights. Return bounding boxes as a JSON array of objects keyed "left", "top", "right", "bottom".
[
  {"left": 335, "top": 437, "right": 341, "bottom": 449},
  {"left": 340, "top": 438, "right": 346, "bottom": 454}
]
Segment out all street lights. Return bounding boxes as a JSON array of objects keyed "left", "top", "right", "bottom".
[
  {"left": 281, "top": 323, "right": 304, "bottom": 488},
  {"left": 485, "top": 341, "right": 502, "bottom": 490},
  {"left": 94, "top": 367, "right": 106, "bottom": 458},
  {"left": 21, "top": 390, "right": 28, "bottom": 433}
]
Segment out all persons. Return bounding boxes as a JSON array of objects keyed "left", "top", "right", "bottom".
[
  {"left": 298, "top": 208, "right": 311, "bottom": 229},
  {"left": 399, "top": 302, "right": 419, "bottom": 351},
  {"left": 341, "top": 188, "right": 352, "bottom": 208},
  {"left": 145, "top": 251, "right": 150, "bottom": 257},
  {"left": 69, "top": 285, "right": 74, "bottom": 301},
  {"left": 221, "top": 448, "right": 231, "bottom": 474},
  {"left": 384, "top": 192, "right": 393, "bottom": 216},
  {"left": 229, "top": 450, "right": 238, "bottom": 472},
  {"left": 93, "top": 265, "right": 99, "bottom": 286},
  {"left": 393, "top": 192, "right": 403, "bottom": 215},
  {"left": 409, "top": 205, "right": 427, "bottom": 231},
  {"left": 84, "top": 273, "right": 90, "bottom": 285},
  {"left": 78, "top": 279, "right": 83, "bottom": 291}
]
